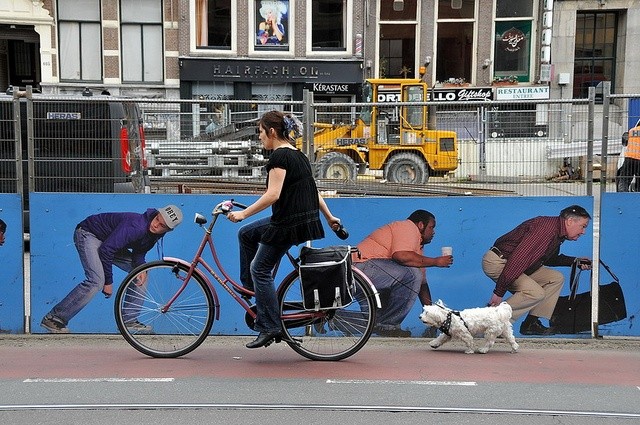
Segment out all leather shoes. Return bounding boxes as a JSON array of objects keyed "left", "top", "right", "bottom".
[{"left": 519, "top": 320, "right": 554, "bottom": 335}]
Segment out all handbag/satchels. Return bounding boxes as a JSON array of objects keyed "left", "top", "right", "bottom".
[{"left": 548, "top": 258, "right": 627, "bottom": 334}]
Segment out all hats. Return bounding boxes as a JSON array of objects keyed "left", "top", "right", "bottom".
[{"left": 158, "top": 205, "right": 183, "bottom": 229}]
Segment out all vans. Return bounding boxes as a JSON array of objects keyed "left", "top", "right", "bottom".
[{"left": 0, "top": 83, "right": 152, "bottom": 195}]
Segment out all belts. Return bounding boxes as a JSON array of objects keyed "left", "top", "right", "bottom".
[{"left": 489, "top": 247, "right": 505, "bottom": 259}]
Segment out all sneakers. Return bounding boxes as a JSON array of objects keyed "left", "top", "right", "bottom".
[
  {"left": 376, "top": 329, "right": 411, "bottom": 337},
  {"left": 40, "top": 316, "right": 70, "bottom": 333},
  {"left": 116, "top": 322, "right": 151, "bottom": 330},
  {"left": 314, "top": 322, "right": 327, "bottom": 334}
]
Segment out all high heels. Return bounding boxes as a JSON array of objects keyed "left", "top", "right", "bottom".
[
  {"left": 246, "top": 328, "right": 282, "bottom": 349},
  {"left": 232, "top": 283, "right": 256, "bottom": 298}
]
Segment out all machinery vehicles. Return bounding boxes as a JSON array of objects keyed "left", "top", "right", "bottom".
[{"left": 294, "top": 77, "right": 458, "bottom": 186}]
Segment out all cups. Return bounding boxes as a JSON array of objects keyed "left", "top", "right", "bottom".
[{"left": 441, "top": 247, "right": 453, "bottom": 256}]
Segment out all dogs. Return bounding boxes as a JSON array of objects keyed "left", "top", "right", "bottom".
[{"left": 419, "top": 298, "right": 520, "bottom": 355}]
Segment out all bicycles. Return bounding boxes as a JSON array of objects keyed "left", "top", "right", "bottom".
[{"left": 114, "top": 201, "right": 381, "bottom": 363}]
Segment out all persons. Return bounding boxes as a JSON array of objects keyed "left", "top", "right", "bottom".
[
  {"left": 257, "top": 2, "right": 285, "bottom": 45},
  {"left": 40, "top": 205, "right": 183, "bottom": 334},
  {"left": 205, "top": 101, "right": 224, "bottom": 132},
  {"left": 226, "top": 110, "right": 340, "bottom": 350},
  {"left": 482, "top": 204, "right": 593, "bottom": 335},
  {"left": 618, "top": 125, "right": 640, "bottom": 192},
  {"left": 314, "top": 210, "right": 438, "bottom": 338},
  {"left": 0, "top": 219, "right": 7, "bottom": 248}
]
